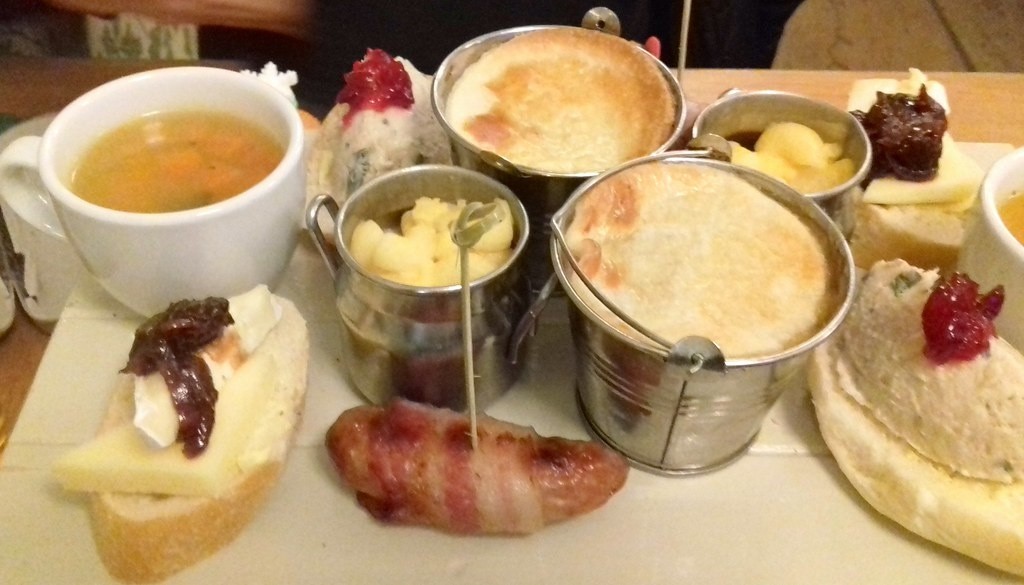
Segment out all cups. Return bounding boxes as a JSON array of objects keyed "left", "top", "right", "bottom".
[
  {"left": 3, "top": 65, "right": 307, "bottom": 325},
  {"left": 961, "top": 145, "right": 1024, "bottom": 355},
  {"left": 307, "top": 160, "right": 560, "bottom": 417}
]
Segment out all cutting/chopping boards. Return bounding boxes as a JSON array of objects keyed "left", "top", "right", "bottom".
[{"left": 3, "top": 222, "right": 1024, "bottom": 585}]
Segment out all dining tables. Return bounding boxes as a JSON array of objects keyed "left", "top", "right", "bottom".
[{"left": 0, "top": 66, "right": 1023, "bottom": 584}]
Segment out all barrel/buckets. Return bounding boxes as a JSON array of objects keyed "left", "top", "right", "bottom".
[
  {"left": 431, "top": 6, "right": 690, "bottom": 299},
  {"left": 548, "top": 133, "right": 859, "bottom": 480}
]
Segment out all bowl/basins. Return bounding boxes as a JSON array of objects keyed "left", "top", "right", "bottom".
[{"left": 695, "top": 86, "right": 873, "bottom": 218}]
